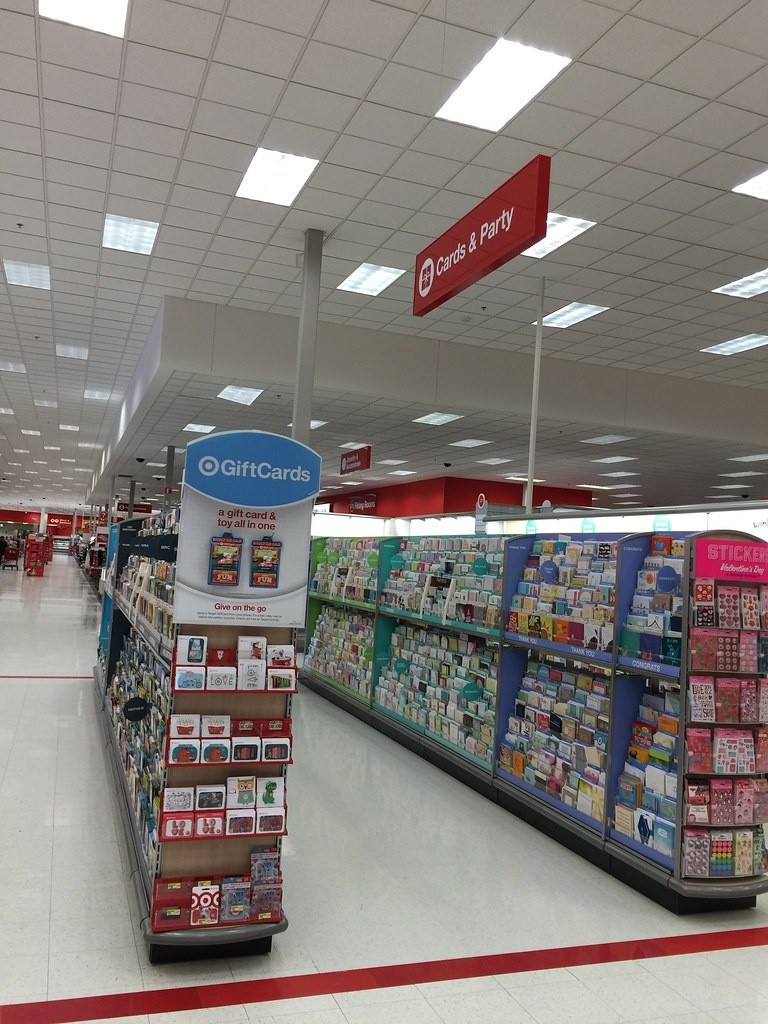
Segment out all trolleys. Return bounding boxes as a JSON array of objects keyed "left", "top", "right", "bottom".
[{"left": 2, "top": 548, "right": 19, "bottom": 571}]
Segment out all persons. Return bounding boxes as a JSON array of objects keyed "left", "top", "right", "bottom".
[
  {"left": 5, "top": 536, "right": 20, "bottom": 548},
  {"left": 22, "top": 530, "right": 26, "bottom": 539},
  {"left": 0, "top": 536, "right": 8, "bottom": 567}
]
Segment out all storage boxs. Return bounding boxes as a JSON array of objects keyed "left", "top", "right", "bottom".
[{"left": 151, "top": 635, "right": 298, "bottom": 933}]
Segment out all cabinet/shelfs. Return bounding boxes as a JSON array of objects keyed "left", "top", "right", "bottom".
[
  {"left": 299, "top": 531, "right": 768, "bottom": 916},
  {"left": 93, "top": 504, "right": 300, "bottom": 963}
]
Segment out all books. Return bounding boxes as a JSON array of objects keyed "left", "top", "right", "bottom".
[{"left": 308, "top": 535, "right": 685, "bottom": 855}]
